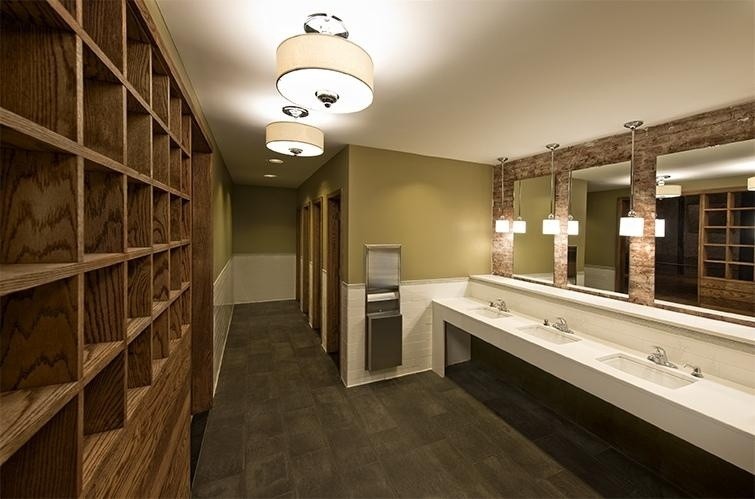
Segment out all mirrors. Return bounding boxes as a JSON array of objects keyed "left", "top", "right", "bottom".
[
  {"left": 512, "top": 174, "right": 555, "bottom": 285},
  {"left": 567, "top": 160, "right": 631, "bottom": 298},
  {"left": 654, "top": 139, "right": 755, "bottom": 317}
]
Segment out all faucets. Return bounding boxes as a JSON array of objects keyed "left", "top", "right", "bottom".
[
  {"left": 489, "top": 296, "right": 508, "bottom": 311},
  {"left": 552, "top": 315, "right": 574, "bottom": 334},
  {"left": 647, "top": 344, "right": 667, "bottom": 366}
]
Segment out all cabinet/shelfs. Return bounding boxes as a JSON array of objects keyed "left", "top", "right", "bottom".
[{"left": 0, "top": 0, "right": 195, "bottom": 498}]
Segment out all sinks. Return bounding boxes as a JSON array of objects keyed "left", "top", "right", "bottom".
[
  {"left": 516, "top": 323, "right": 582, "bottom": 346},
  {"left": 594, "top": 351, "right": 700, "bottom": 393},
  {"left": 469, "top": 307, "right": 513, "bottom": 320}
]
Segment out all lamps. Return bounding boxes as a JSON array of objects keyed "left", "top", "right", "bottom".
[
  {"left": 542, "top": 143, "right": 559, "bottom": 235},
  {"left": 619, "top": 122, "right": 644, "bottom": 237},
  {"left": 265, "top": 106, "right": 325, "bottom": 158},
  {"left": 513, "top": 180, "right": 526, "bottom": 234},
  {"left": 495, "top": 157, "right": 509, "bottom": 232},
  {"left": 655, "top": 176, "right": 682, "bottom": 201},
  {"left": 276, "top": 13, "right": 374, "bottom": 114},
  {"left": 567, "top": 171, "right": 579, "bottom": 236}
]
